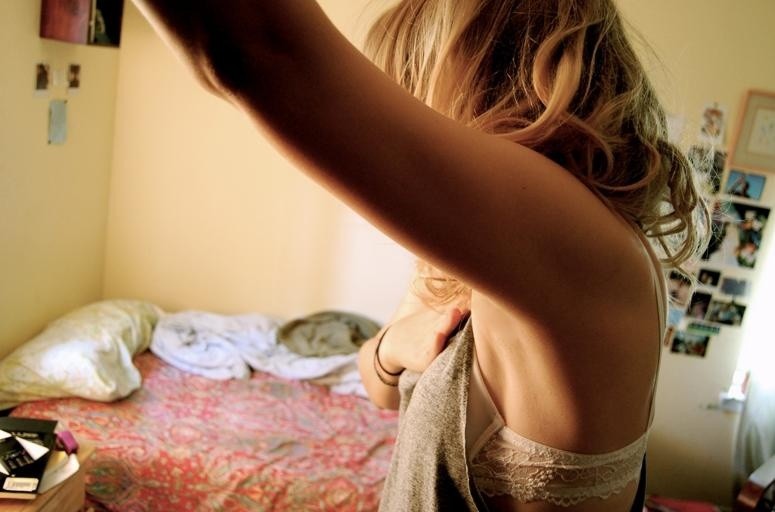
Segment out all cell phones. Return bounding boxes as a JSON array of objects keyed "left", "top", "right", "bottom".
[{"left": 0, "top": 437, "right": 36, "bottom": 474}]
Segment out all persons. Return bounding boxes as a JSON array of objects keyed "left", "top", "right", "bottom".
[{"left": 727, "top": 173, "right": 749, "bottom": 198}]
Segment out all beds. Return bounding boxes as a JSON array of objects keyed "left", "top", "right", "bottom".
[{"left": 2, "top": 324, "right": 399, "bottom": 510}]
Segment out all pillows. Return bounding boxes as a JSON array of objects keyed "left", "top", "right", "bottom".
[{"left": 6, "top": 296, "right": 164, "bottom": 400}]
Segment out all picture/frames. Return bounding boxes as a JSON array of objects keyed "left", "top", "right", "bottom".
[{"left": 722, "top": 85, "right": 775, "bottom": 179}]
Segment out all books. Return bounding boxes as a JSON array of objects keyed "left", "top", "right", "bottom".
[{"left": 0, "top": 417, "right": 58, "bottom": 499}]
